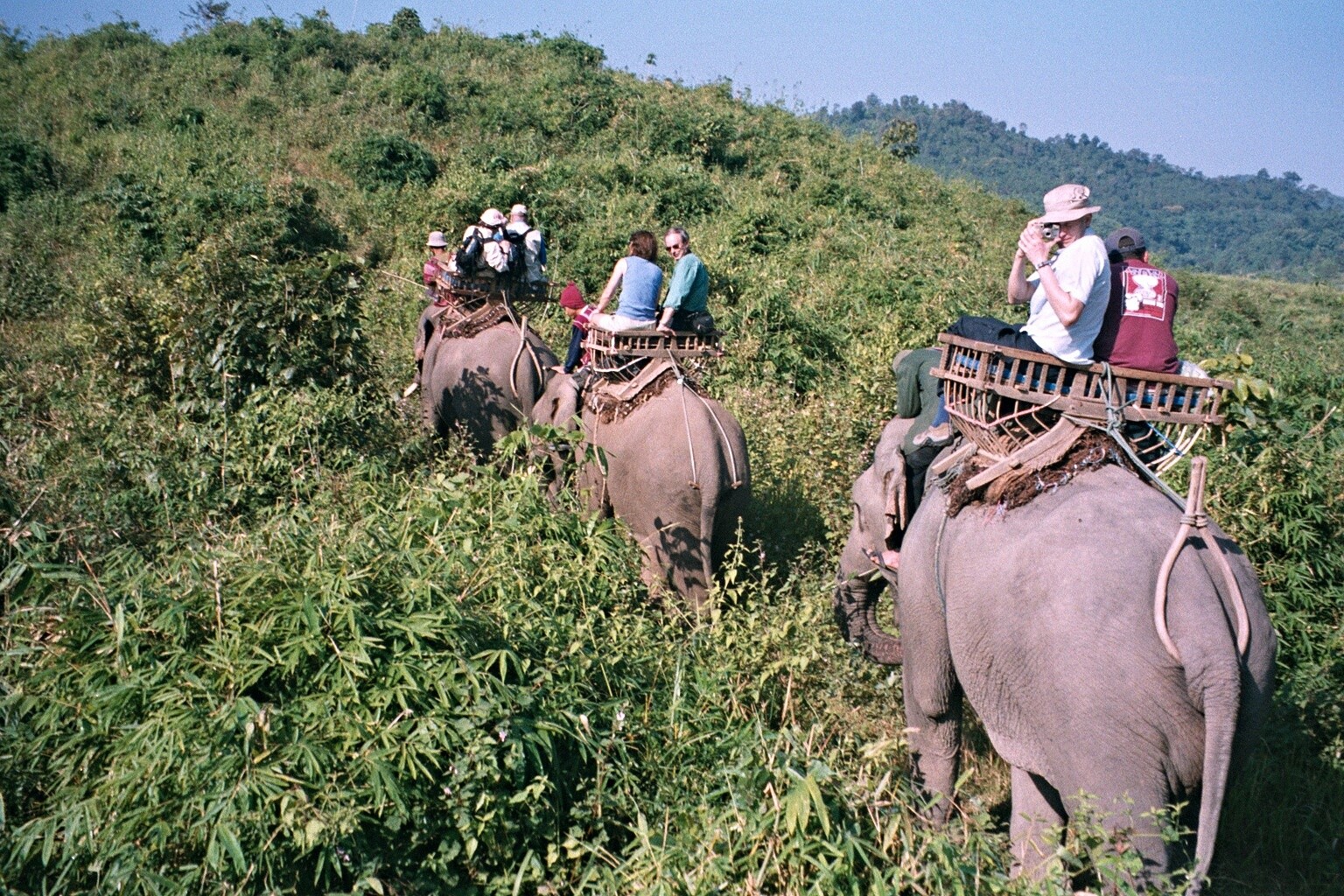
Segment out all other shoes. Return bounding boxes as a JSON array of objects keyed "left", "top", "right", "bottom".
[{"left": 415, "top": 350, "right": 424, "bottom": 361}]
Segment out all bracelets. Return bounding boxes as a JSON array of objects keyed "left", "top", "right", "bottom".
[{"left": 1036, "top": 261, "right": 1053, "bottom": 269}]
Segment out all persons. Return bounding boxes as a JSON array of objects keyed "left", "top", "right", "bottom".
[
  {"left": 550, "top": 282, "right": 604, "bottom": 374},
  {"left": 498, "top": 203, "right": 548, "bottom": 292},
  {"left": 460, "top": 208, "right": 511, "bottom": 291},
  {"left": 423, "top": 231, "right": 460, "bottom": 287},
  {"left": 892, "top": 348, "right": 987, "bottom": 555},
  {"left": 912, "top": 182, "right": 1111, "bottom": 446},
  {"left": 587, "top": 230, "right": 663, "bottom": 340},
  {"left": 656, "top": 226, "right": 709, "bottom": 337},
  {"left": 1104, "top": 227, "right": 1179, "bottom": 394}
]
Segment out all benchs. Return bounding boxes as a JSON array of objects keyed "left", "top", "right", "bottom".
[
  {"left": 929, "top": 333, "right": 1237, "bottom": 426},
  {"left": 442, "top": 271, "right": 560, "bottom": 302},
  {"left": 580, "top": 323, "right": 730, "bottom": 358}
]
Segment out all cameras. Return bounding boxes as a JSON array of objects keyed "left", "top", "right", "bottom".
[{"left": 1040, "top": 224, "right": 1059, "bottom": 241}]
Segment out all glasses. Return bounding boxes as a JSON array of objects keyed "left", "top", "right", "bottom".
[{"left": 665, "top": 245, "right": 679, "bottom": 252}]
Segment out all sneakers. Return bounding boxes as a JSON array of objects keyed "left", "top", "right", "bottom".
[{"left": 912, "top": 422, "right": 953, "bottom": 447}]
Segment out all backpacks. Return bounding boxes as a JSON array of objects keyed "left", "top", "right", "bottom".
[
  {"left": 502, "top": 227, "right": 535, "bottom": 277},
  {"left": 456, "top": 229, "right": 496, "bottom": 277}
]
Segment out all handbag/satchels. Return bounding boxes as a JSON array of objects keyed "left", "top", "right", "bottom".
[{"left": 681, "top": 310, "right": 714, "bottom": 334}]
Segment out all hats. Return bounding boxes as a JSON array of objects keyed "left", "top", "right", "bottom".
[
  {"left": 893, "top": 350, "right": 913, "bottom": 372},
  {"left": 1039, "top": 184, "right": 1100, "bottom": 223},
  {"left": 510, "top": 204, "right": 527, "bottom": 215},
  {"left": 560, "top": 281, "right": 585, "bottom": 309},
  {"left": 480, "top": 208, "right": 507, "bottom": 225},
  {"left": 1105, "top": 228, "right": 1146, "bottom": 255},
  {"left": 426, "top": 231, "right": 448, "bottom": 246}
]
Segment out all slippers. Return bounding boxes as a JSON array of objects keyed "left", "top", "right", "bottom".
[{"left": 860, "top": 547, "right": 898, "bottom": 577}]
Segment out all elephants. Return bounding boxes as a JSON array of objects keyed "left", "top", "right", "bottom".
[
  {"left": 414, "top": 303, "right": 560, "bottom": 473},
  {"left": 832, "top": 403, "right": 1276, "bottom": 896},
  {"left": 526, "top": 371, "right": 753, "bottom": 635}
]
